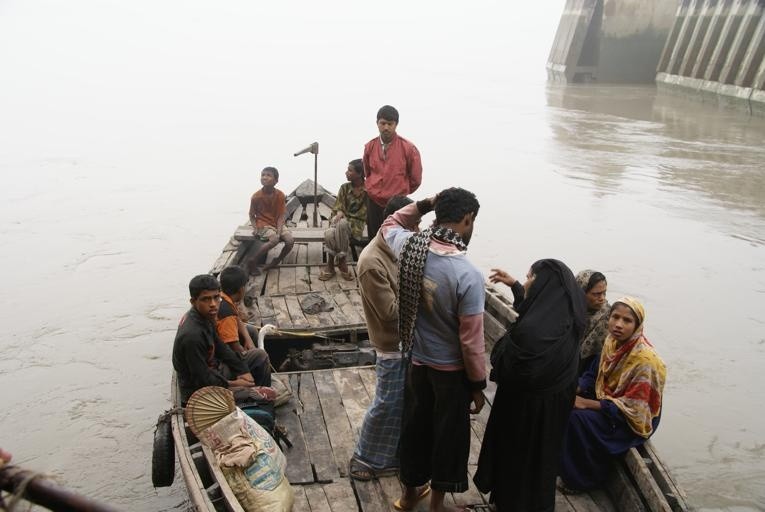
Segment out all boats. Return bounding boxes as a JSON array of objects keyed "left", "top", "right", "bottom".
[{"left": 170, "top": 179, "right": 690, "bottom": 511}]
